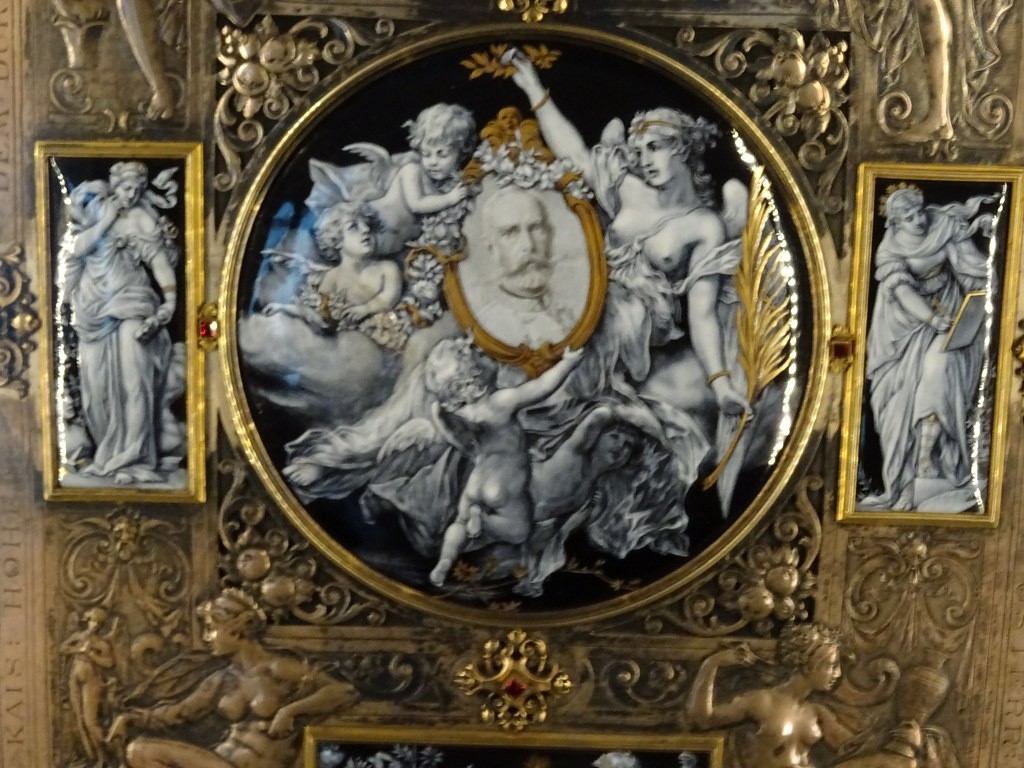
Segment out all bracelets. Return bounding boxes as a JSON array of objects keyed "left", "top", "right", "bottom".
[
  {"left": 926, "top": 309, "right": 940, "bottom": 326},
  {"left": 707, "top": 370, "right": 733, "bottom": 388},
  {"left": 163, "top": 285, "right": 177, "bottom": 293}
]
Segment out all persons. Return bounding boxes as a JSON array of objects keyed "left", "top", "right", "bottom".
[
  {"left": 61, "top": 162, "right": 177, "bottom": 484},
  {"left": 57, "top": 606, "right": 116, "bottom": 767},
  {"left": 687, "top": 621, "right": 926, "bottom": 768},
  {"left": 105, "top": 586, "right": 362, "bottom": 768},
  {"left": 235, "top": 49, "right": 752, "bottom": 586},
  {"left": 865, "top": 181, "right": 998, "bottom": 515}
]
